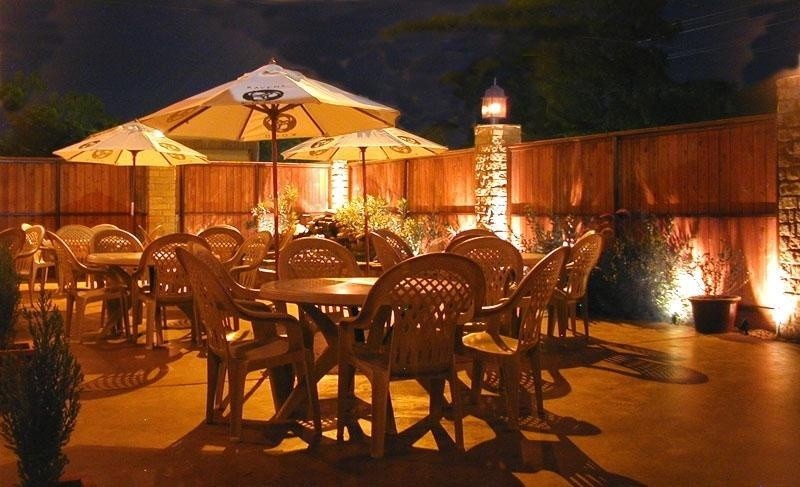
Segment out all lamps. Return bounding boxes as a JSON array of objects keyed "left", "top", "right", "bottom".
[{"left": 482, "top": 76, "right": 506, "bottom": 124}]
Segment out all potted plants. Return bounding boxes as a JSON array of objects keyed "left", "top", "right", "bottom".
[
  {"left": 0, "top": 243, "right": 34, "bottom": 361},
  {"left": 688, "top": 237, "right": 750, "bottom": 334},
  {"left": 0, "top": 282, "right": 91, "bottom": 487}
]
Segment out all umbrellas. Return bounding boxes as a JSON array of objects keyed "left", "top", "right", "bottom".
[
  {"left": 280, "top": 127, "right": 449, "bottom": 277},
  {"left": 135, "top": 58, "right": 401, "bottom": 281},
  {"left": 53, "top": 118, "right": 211, "bottom": 236}
]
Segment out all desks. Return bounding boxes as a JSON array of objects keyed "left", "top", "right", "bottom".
[
  {"left": 261, "top": 277, "right": 468, "bottom": 439},
  {"left": 88, "top": 252, "right": 220, "bottom": 345}
]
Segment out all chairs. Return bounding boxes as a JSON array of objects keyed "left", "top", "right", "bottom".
[
  {"left": 0, "top": 227, "right": 26, "bottom": 260},
  {"left": 192, "top": 244, "right": 260, "bottom": 300},
  {"left": 54, "top": 225, "right": 96, "bottom": 295},
  {"left": 336, "top": 253, "right": 485, "bottom": 459},
  {"left": 9, "top": 224, "right": 45, "bottom": 308},
  {"left": 370, "top": 232, "right": 401, "bottom": 272},
  {"left": 92, "top": 224, "right": 119, "bottom": 231},
  {"left": 461, "top": 246, "right": 571, "bottom": 419},
  {"left": 142, "top": 225, "right": 163, "bottom": 246},
  {"left": 90, "top": 229, "right": 145, "bottom": 328},
  {"left": 21, "top": 223, "right": 55, "bottom": 292},
  {"left": 278, "top": 237, "right": 362, "bottom": 279},
  {"left": 451, "top": 236, "right": 524, "bottom": 305},
  {"left": 221, "top": 231, "right": 273, "bottom": 289},
  {"left": 175, "top": 246, "right": 322, "bottom": 440},
  {"left": 547, "top": 233, "right": 606, "bottom": 349},
  {"left": 575, "top": 229, "right": 595, "bottom": 248},
  {"left": 130, "top": 233, "right": 212, "bottom": 350},
  {"left": 137, "top": 224, "right": 153, "bottom": 244},
  {"left": 44, "top": 230, "right": 131, "bottom": 346},
  {"left": 196, "top": 224, "right": 246, "bottom": 330},
  {"left": 374, "top": 229, "right": 414, "bottom": 260}
]
